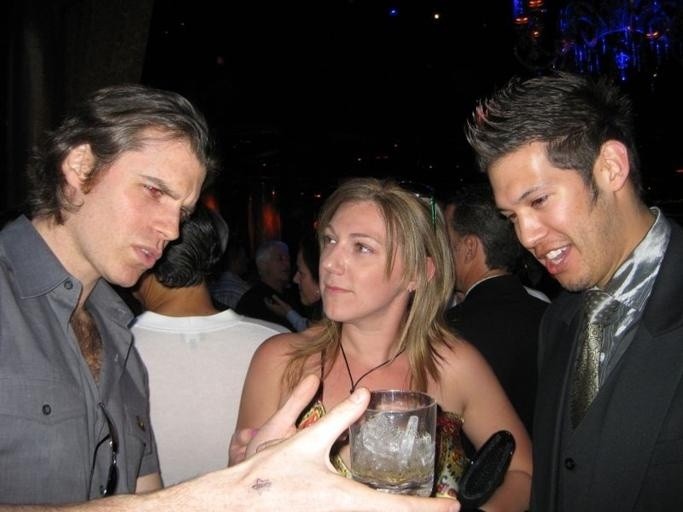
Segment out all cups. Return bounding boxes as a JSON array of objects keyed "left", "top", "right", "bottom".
[{"left": 347, "top": 389, "right": 436, "bottom": 499}]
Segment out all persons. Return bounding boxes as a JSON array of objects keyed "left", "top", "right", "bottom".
[
  {"left": 1, "top": 82, "right": 461, "bottom": 512},
  {"left": 125, "top": 194, "right": 554, "bottom": 488},
  {"left": 227, "top": 175, "right": 537, "bottom": 512},
  {"left": 467, "top": 70, "right": 682, "bottom": 510}
]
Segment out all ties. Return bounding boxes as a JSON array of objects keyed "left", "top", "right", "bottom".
[{"left": 568, "top": 289, "right": 620, "bottom": 431}]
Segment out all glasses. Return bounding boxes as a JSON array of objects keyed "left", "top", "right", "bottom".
[{"left": 85, "top": 401, "right": 119, "bottom": 501}]
